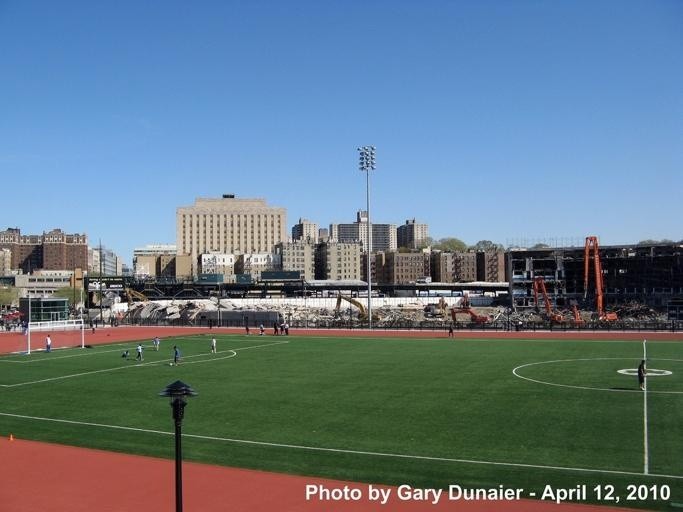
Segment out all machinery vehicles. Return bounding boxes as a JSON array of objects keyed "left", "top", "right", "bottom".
[
  {"left": 582, "top": 234, "right": 618, "bottom": 321},
  {"left": 334, "top": 294, "right": 381, "bottom": 321},
  {"left": 423, "top": 297, "right": 447, "bottom": 319},
  {"left": 449, "top": 306, "right": 490, "bottom": 324},
  {"left": 124, "top": 286, "right": 150, "bottom": 307},
  {"left": 532, "top": 276, "right": 568, "bottom": 321}
]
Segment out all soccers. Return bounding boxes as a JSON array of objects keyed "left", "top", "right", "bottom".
[{"left": 170, "top": 363, "right": 173, "bottom": 366}]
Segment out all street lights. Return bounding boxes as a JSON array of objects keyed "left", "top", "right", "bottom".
[{"left": 156, "top": 379, "right": 201, "bottom": 512}]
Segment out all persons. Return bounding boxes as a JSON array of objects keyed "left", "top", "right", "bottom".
[
  {"left": 121, "top": 351, "right": 130, "bottom": 358},
  {"left": 152, "top": 336, "right": 160, "bottom": 351},
  {"left": 136, "top": 344, "right": 143, "bottom": 362},
  {"left": 636, "top": 358, "right": 647, "bottom": 392},
  {"left": 42, "top": 334, "right": 53, "bottom": 352},
  {"left": 174, "top": 345, "right": 180, "bottom": 366},
  {"left": 447, "top": 321, "right": 455, "bottom": 339},
  {"left": 246, "top": 319, "right": 290, "bottom": 336},
  {"left": 210, "top": 337, "right": 217, "bottom": 354}
]
